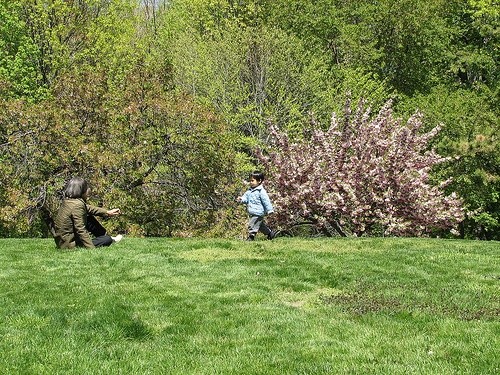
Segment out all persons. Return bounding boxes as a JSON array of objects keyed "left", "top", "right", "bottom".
[
  {"left": 236, "top": 171, "right": 276, "bottom": 242},
  {"left": 54, "top": 176, "right": 123, "bottom": 250}
]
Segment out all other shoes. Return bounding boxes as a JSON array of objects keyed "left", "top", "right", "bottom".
[
  {"left": 267, "top": 232, "right": 276, "bottom": 240},
  {"left": 245, "top": 236, "right": 254, "bottom": 241},
  {"left": 110, "top": 234, "right": 122, "bottom": 246}
]
